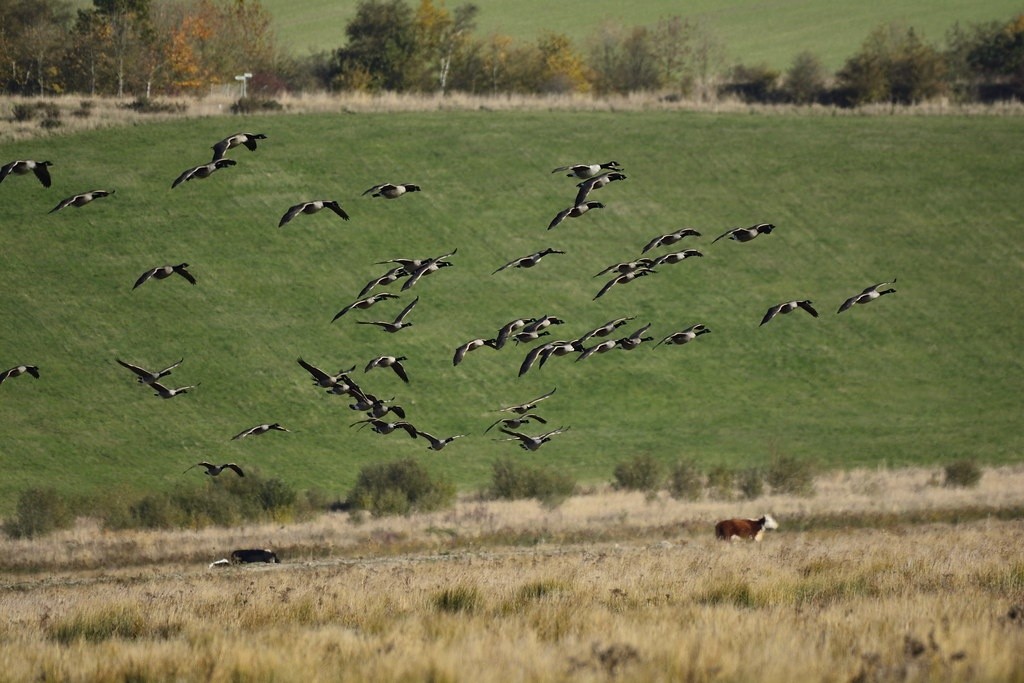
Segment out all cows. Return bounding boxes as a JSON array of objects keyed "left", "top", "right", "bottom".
[
  {"left": 715, "top": 513, "right": 779, "bottom": 542},
  {"left": 231, "top": 548, "right": 280, "bottom": 565}
]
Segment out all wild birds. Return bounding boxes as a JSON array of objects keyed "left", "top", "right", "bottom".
[
  {"left": 231, "top": 423, "right": 291, "bottom": 441},
  {"left": 182, "top": 462, "right": 245, "bottom": 478},
  {"left": 132, "top": 263, "right": 197, "bottom": 290},
  {"left": 490, "top": 161, "right": 775, "bottom": 301},
  {"left": 331, "top": 249, "right": 457, "bottom": 333},
  {"left": 47, "top": 189, "right": 115, "bottom": 215},
  {"left": 482, "top": 387, "right": 570, "bottom": 452},
  {"left": 172, "top": 158, "right": 237, "bottom": 188},
  {"left": 361, "top": 183, "right": 421, "bottom": 199},
  {"left": 278, "top": 200, "right": 350, "bottom": 229},
  {"left": 0, "top": 160, "right": 53, "bottom": 188},
  {"left": 297, "top": 355, "right": 471, "bottom": 451},
  {"left": 452, "top": 314, "right": 711, "bottom": 378},
  {"left": 114, "top": 355, "right": 183, "bottom": 384},
  {"left": 211, "top": 132, "right": 267, "bottom": 161},
  {"left": 759, "top": 277, "right": 898, "bottom": 328},
  {"left": 146, "top": 382, "right": 201, "bottom": 400},
  {"left": 0, "top": 365, "right": 40, "bottom": 384}
]
